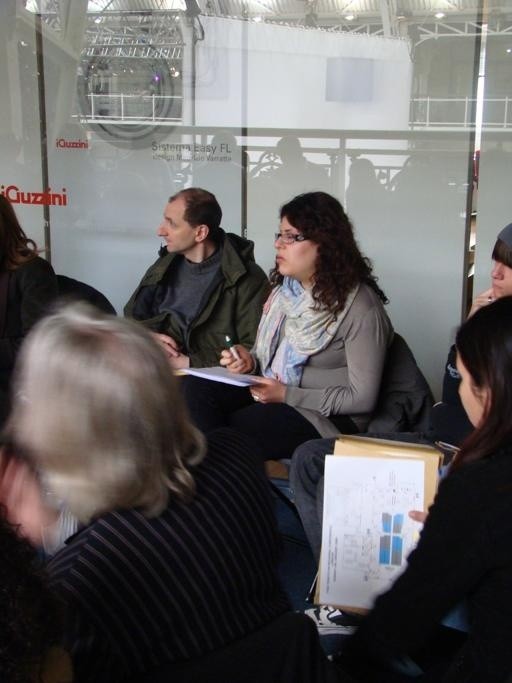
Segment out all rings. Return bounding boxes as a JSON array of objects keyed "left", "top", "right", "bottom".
[
  {"left": 487, "top": 296, "right": 492, "bottom": 303},
  {"left": 253, "top": 395, "right": 260, "bottom": 401}
]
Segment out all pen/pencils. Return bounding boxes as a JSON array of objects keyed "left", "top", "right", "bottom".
[{"left": 225, "top": 335, "right": 241, "bottom": 360}]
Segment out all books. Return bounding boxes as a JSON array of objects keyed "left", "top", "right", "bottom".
[{"left": 177, "top": 365, "right": 262, "bottom": 387}]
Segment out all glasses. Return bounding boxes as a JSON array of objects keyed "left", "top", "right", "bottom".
[{"left": 275, "top": 233, "right": 311, "bottom": 244}]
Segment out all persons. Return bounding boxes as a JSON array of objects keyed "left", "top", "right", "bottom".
[
  {"left": 332, "top": 295, "right": 511, "bottom": 682},
  {"left": 288, "top": 222, "right": 511, "bottom": 635},
  {"left": 2, "top": 195, "right": 58, "bottom": 416},
  {"left": 179, "top": 193, "right": 397, "bottom": 465},
  {"left": 2, "top": 301, "right": 289, "bottom": 681},
  {"left": 121, "top": 186, "right": 270, "bottom": 371}
]
